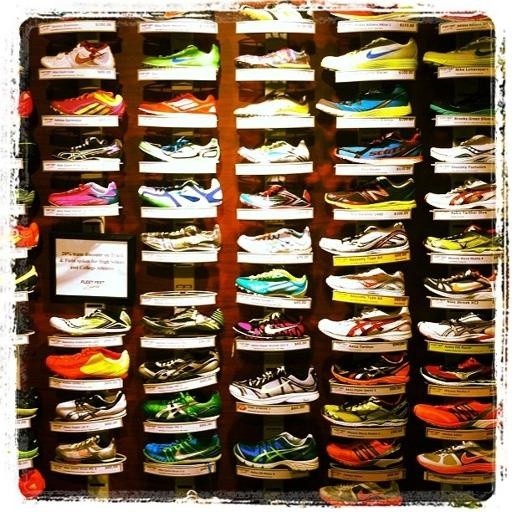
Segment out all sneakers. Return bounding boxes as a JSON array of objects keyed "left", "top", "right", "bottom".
[{"left": 16, "top": 4, "right": 504, "bottom": 506}]
